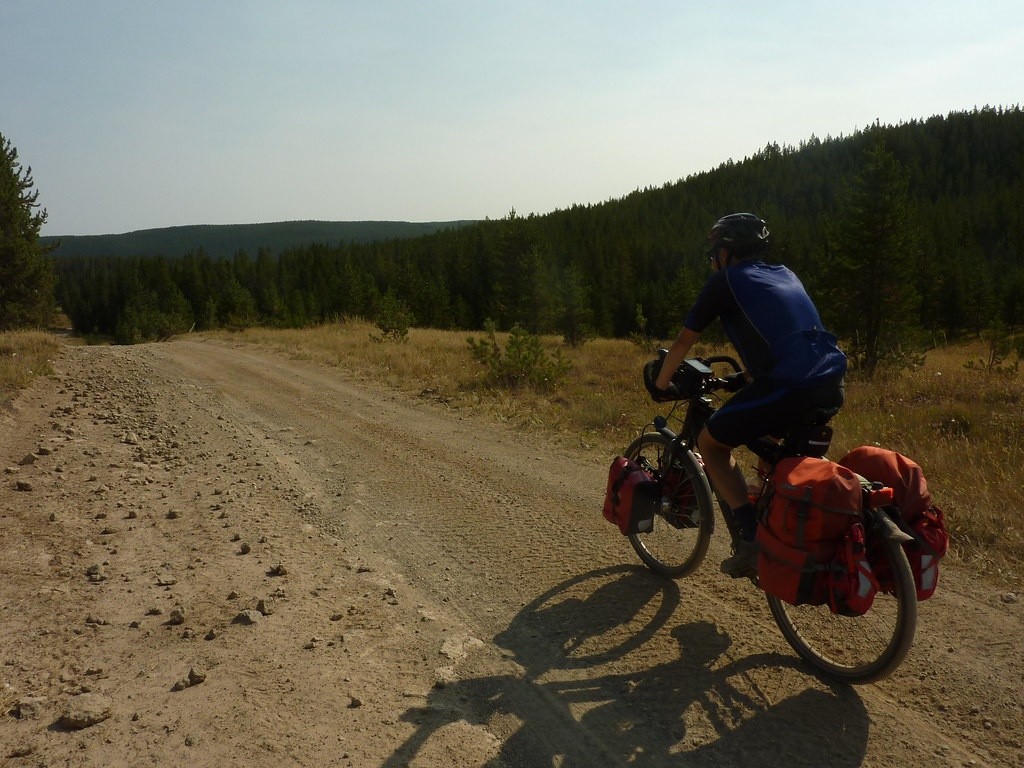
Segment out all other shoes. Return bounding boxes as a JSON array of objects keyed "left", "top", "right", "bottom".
[{"left": 720, "top": 539, "right": 758, "bottom": 576}]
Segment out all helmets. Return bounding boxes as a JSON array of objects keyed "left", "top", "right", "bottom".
[{"left": 709, "top": 212, "right": 771, "bottom": 253}]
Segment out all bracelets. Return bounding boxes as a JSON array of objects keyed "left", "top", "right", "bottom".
[{"left": 661, "top": 354, "right": 684, "bottom": 373}]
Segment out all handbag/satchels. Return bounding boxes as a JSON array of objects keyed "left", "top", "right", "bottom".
[
  {"left": 602, "top": 456, "right": 658, "bottom": 536},
  {"left": 653, "top": 452, "right": 713, "bottom": 530},
  {"left": 751, "top": 456, "right": 880, "bottom": 617},
  {"left": 837, "top": 446, "right": 950, "bottom": 601}
]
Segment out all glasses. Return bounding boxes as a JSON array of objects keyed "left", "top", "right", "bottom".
[{"left": 705, "top": 243, "right": 723, "bottom": 263}]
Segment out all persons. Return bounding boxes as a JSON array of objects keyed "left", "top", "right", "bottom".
[{"left": 653, "top": 214, "right": 847, "bottom": 580}]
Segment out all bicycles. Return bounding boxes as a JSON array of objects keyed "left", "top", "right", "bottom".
[{"left": 610, "top": 346, "right": 919, "bottom": 687}]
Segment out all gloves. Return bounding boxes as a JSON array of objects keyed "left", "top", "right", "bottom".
[
  {"left": 651, "top": 379, "right": 679, "bottom": 402},
  {"left": 723, "top": 370, "right": 750, "bottom": 394}
]
